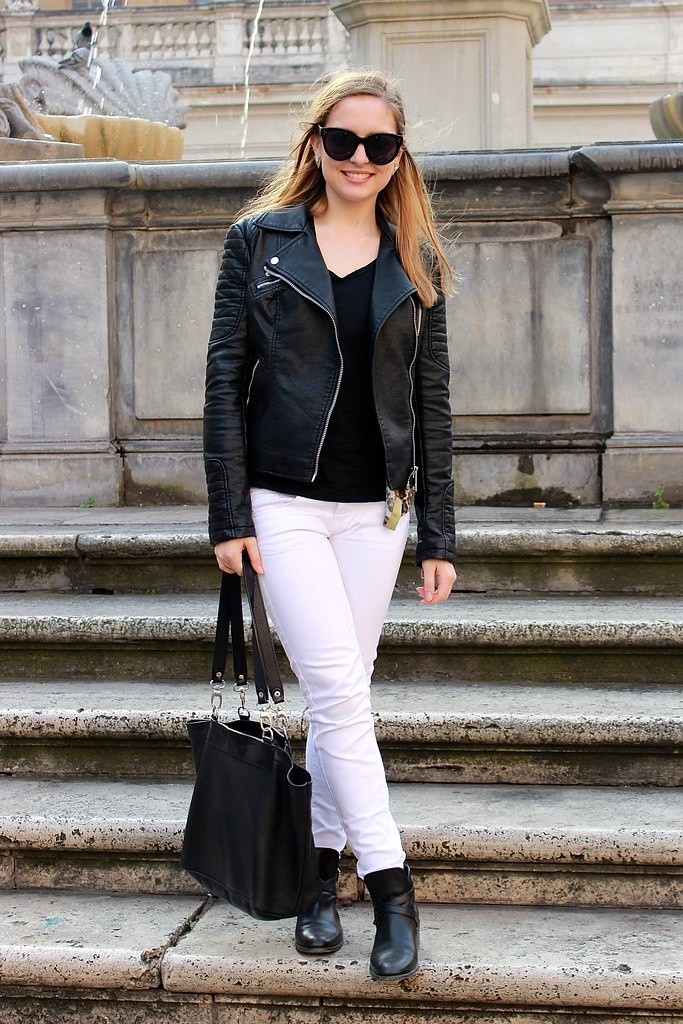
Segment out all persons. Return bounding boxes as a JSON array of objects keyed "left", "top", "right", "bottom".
[{"left": 202, "top": 71, "right": 458, "bottom": 980}]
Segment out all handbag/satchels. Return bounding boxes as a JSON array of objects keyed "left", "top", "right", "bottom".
[{"left": 179, "top": 551, "right": 320, "bottom": 921}]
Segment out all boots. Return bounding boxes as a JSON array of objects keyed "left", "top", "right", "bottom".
[
  {"left": 357, "top": 862, "right": 420, "bottom": 980},
  {"left": 295, "top": 846, "right": 344, "bottom": 954}
]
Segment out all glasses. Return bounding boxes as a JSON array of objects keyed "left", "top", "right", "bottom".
[{"left": 318, "top": 125, "right": 403, "bottom": 165}]
